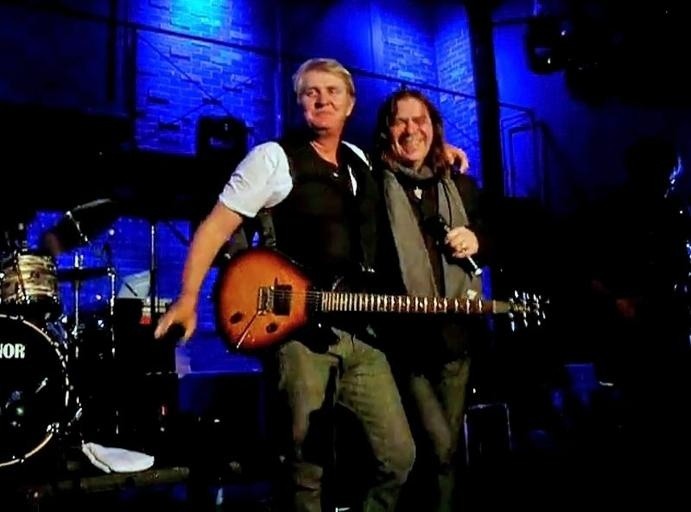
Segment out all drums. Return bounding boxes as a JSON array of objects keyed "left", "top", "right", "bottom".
[
  {"left": 1, "top": 248, "right": 62, "bottom": 329},
  {"left": 0, "top": 314, "right": 82, "bottom": 465}
]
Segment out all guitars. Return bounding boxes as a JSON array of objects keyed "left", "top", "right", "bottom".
[{"left": 213, "top": 246, "right": 549, "bottom": 356}]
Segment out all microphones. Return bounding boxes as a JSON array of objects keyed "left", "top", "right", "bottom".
[{"left": 426, "top": 213, "right": 482, "bottom": 276}]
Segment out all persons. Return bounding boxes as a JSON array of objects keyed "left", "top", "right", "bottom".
[
  {"left": 152, "top": 57, "right": 472, "bottom": 511},
  {"left": 371, "top": 84, "right": 513, "bottom": 512}
]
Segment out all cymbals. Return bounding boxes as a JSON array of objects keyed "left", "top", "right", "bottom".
[
  {"left": 53, "top": 198, "right": 116, "bottom": 248},
  {"left": 59, "top": 266, "right": 106, "bottom": 282}
]
